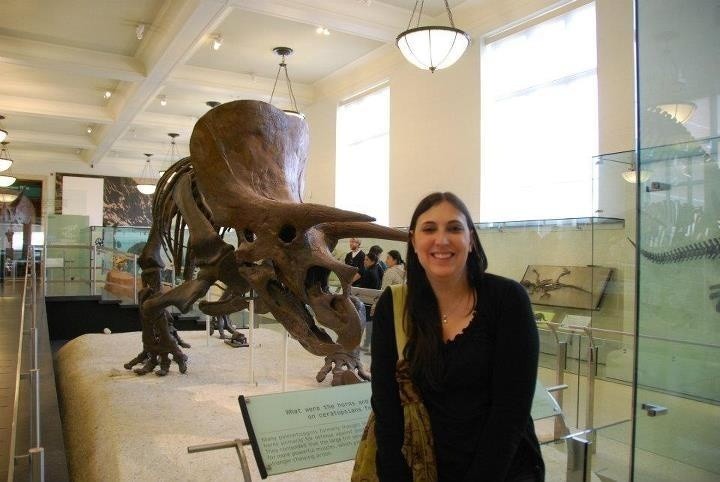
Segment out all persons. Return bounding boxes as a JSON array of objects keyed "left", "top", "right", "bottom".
[
  {"left": 369, "top": 250, "right": 408, "bottom": 318},
  {"left": 352, "top": 252, "right": 384, "bottom": 350},
  {"left": 369, "top": 191, "right": 547, "bottom": 482},
  {"left": 368, "top": 246, "right": 388, "bottom": 271},
  {"left": 344, "top": 237, "right": 364, "bottom": 269}
]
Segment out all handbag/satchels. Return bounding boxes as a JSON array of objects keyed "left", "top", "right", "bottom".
[{"left": 351, "top": 359, "right": 437, "bottom": 482}]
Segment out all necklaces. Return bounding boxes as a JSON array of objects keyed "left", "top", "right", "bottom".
[{"left": 441, "top": 294, "right": 464, "bottom": 325}]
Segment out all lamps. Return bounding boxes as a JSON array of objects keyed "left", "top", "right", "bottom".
[
  {"left": 137, "top": 133, "right": 180, "bottom": 195},
  {"left": 395, "top": 1, "right": 472, "bottom": 76},
  {"left": 268, "top": 47, "right": 306, "bottom": 123},
  {"left": 0, "top": 115, "right": 19, "bottom": 203},
  {"left": 622, "top": 152, "right": 651, "bottom": 185},
  {"left": 648, "top": 56, "right": 696, "bottom": 127}
]
